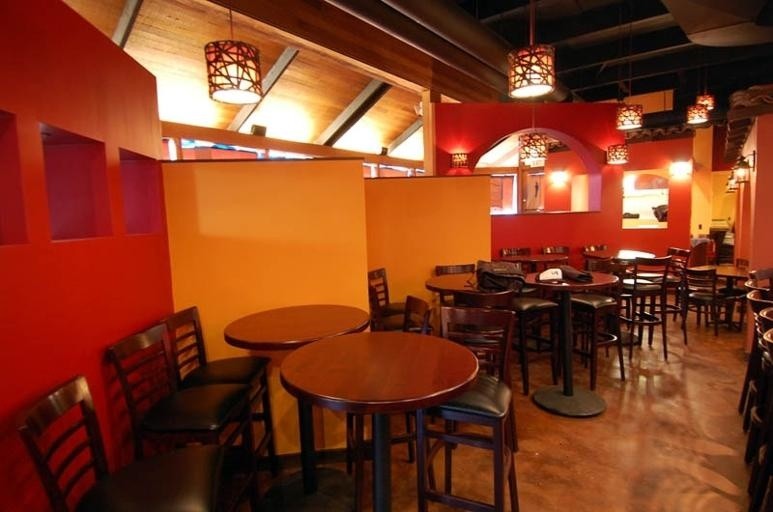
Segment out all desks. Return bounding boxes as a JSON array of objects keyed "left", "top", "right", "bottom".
[
  {"left": 280, "top": 331, "right": 479, "bottom": 511},
  {"left": 224, "top": 304, "right": 372, "bottom": 494}
]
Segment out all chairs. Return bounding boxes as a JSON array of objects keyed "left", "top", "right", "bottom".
[
  {"left": 160, "top": 306, "right": 276, "bottom": 469},
  {"left": 18, "top": 375, "right": 225, "bottom": 512},
  {"left": 106, "top": 323, "right": 252, "bottom": 478}
]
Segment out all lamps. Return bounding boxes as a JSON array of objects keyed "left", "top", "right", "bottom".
[
  {"left": 518, "top": 103, "right": 549, "bottom": 160},
  {"left": 685, "top": 92, "right": 715, "bottom": 124},
  {"left": 607, "top": 144, "right": 628, "bottom": 165},
  {"left": 724, "top": 150, "right": 756, "bottom": 194},
  {"left": 508, "top": 4, "right": 556, "bottom": 97},
  {"left": 616, "top": 66, "right": 642, "bottom": 130},
  {"left": 450, "top": 153, "right": 469, "bottom": 172},
  {"left": 205, "top": 4, "right": 263, "bottom": 104}
]
